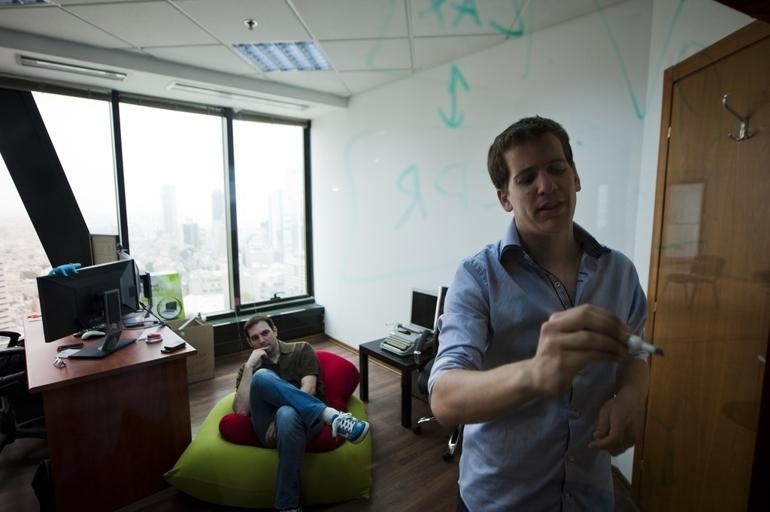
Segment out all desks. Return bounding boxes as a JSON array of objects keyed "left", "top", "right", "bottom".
[
  {"left": 360, "top": 331, "right": 435, "bottom": 427},
  {"left": 23, "top": 315, "right": 199, "bottom": 512}
]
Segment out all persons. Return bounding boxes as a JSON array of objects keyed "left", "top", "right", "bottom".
[
  {"left": 427, "top": 114, "right": 649, "bottom": 512},
  {"left": 233, "top": 316, "right": 369, "bottom": 512}
]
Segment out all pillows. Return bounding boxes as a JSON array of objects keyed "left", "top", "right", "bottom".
[{"left": 220, "top": 352, "right": 359, "bottom": 450}]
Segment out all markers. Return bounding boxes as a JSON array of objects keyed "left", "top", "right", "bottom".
[{"left": 623, "top": 334, "right": 665, "bottom": 356}]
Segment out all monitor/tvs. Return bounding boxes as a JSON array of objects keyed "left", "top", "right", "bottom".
[
  {"left": 411, "top": 292, "right": 437, "bottom": 334},
  {"left": 437, "top": 287, "right": 447, "bottom": 328},
  {"left": 34, "top": 233, "right": 151, "bottom": 360}
]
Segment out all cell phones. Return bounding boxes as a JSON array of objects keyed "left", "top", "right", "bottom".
[{"left": 164, "top": 342, "right": 185, "bottom": 350}]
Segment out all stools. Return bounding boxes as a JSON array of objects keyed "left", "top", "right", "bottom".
[{"left": 165, "top": 392, "right": 371, "bottom": 508}]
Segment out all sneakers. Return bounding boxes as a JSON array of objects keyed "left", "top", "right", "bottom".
[{"left": 331, "top": 410, "right": 372, "bottom": 445}]
